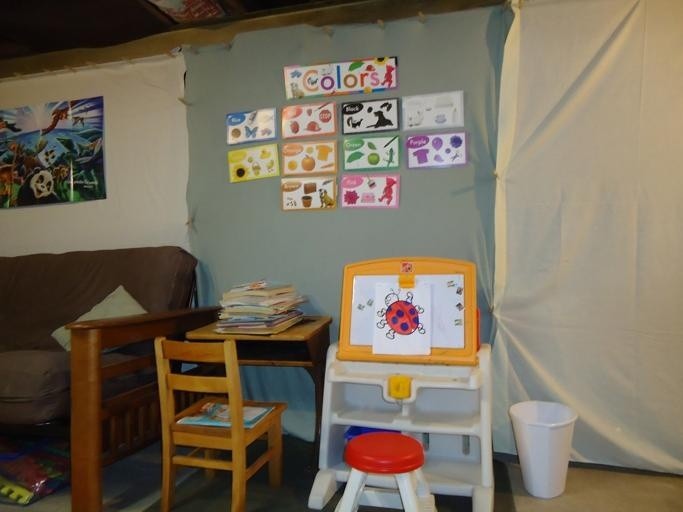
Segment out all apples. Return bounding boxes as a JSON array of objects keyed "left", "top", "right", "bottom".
[{"left": 302, "top": 154, "right": 315, "bottom": 171}]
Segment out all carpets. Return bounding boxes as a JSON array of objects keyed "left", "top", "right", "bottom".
[{"left": 0, "top": 430, "right": 515, "bottom": 511}]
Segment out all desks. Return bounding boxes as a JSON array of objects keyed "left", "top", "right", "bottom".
[{"left": 184, "top": 314, "right": 332, "bottom": 466}]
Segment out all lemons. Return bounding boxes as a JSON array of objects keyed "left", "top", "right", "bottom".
[{"left": 368, "top": 153, "right": 380, "bottom": 164}]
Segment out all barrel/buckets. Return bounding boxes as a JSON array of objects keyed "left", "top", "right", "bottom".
[{"left": 507, "top": 399, "right": 576, "bottom": 500}]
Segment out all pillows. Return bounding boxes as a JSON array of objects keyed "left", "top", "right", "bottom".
[{"left": 48, "top": 283, "right": 148, "bottom": 354}]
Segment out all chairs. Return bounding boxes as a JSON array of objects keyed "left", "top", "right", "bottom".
[{"left": 152, "top": 335, "right": 287, "bottom": 511}]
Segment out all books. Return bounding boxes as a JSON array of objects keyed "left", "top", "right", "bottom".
[
  {"left": 176, "top": 401, "right": 276, "bottom": 429},
  {"left": 215, "top": 282, "right": 304, "bottom": 335}
]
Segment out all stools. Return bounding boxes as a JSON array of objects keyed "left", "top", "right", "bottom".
[{"left": 330, "top": 431, "right": 437, "bottom": 512}]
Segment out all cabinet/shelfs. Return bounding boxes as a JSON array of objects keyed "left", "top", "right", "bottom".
[{"left": 308, "top": 340, "right": 495, "bottom": 511}]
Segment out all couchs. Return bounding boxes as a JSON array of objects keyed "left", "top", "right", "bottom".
[{"left": 0, "top": 245, "right": 198, "bottom": 438}]
